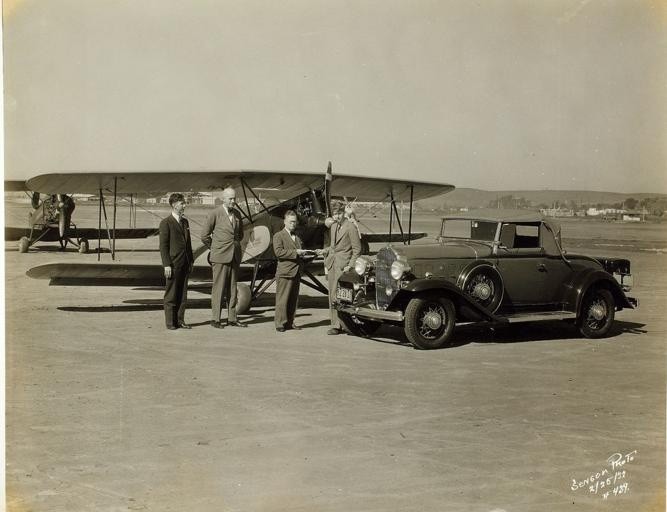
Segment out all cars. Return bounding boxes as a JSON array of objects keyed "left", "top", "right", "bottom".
[{"left": 331, "top": 216, "right": 641, "bottom": 351}]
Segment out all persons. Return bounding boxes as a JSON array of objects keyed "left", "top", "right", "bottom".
[
  {"left": 158, "top": 193, "right": 195, "bottom": 331},
  {"left": 271, "top": 209, "right": 306, "bottom": 332},
  {"left": 200, "top": 188, "right": 247, "bottom": 330},
  {"left": 315, "top": 200, "right": 364, "bottom": 335}
]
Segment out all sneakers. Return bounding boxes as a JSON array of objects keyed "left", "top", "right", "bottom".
[{"left": 326, "top": 328, "right": 345, "bottom": 335}]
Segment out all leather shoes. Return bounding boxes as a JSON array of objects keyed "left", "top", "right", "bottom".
[
  {"left": 165, "top": 322, "right": 191, "bottom": 331},
  {"left": 274, "top": 323, "right": 301, "bottom": 333},
  {"left": 210, "top": 320, "right": 224, "bottom": 329},
  {"left": 228, "top": 320, "right": 247, "bottom": 328}
]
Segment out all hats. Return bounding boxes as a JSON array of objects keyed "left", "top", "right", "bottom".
[{"left": 331, "top": 201, "right": 346, "bottom": 212}]
